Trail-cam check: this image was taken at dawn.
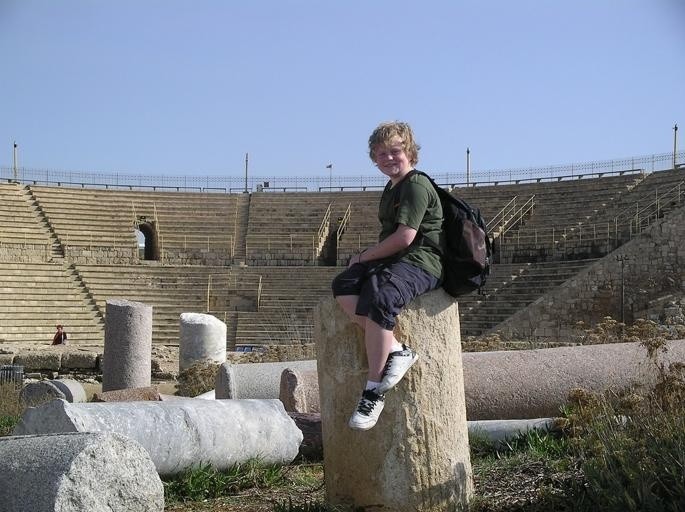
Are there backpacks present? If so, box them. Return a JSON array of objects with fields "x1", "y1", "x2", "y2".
[{"x1": 394, "y1": 170, "x2": 495, "y2": 298}]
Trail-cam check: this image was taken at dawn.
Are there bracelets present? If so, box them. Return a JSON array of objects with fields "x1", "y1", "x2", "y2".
[{"x1": 358, "y1": 255, "x2": 360, "y2": 264}]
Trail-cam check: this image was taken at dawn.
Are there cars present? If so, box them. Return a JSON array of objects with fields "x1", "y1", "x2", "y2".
[{"x1": 234, "y1": 343, "x2": 261, "y2": 353}]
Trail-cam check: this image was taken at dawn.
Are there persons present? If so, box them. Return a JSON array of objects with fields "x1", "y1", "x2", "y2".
[
  {"x1": 330, "y1": 118, "x2": 445, "y2": 429},
  {"x1": 51, "y1": 324, "x2": 67, "y2": 346}
]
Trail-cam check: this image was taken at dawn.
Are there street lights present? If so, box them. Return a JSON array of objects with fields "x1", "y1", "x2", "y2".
[
  {"x1": 673, "y1": 124, "x2": 678, "y2": 168},
  {"x1": 325, "y1": 163, "x2": 332, "y2": 192},
  {"x1": 466, "y1": 148, "x2": 470, "y2": 184},
  {"x1": 12, "y1": 142, "x2": 19, "y2": 181}
]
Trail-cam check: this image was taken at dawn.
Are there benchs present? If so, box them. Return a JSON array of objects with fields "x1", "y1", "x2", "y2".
[{"x1": 0, "y1": 167, "x2": 684, "y2": 351}]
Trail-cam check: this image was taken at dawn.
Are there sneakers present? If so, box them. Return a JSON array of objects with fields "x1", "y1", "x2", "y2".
[
  {"x1": 349, "y1": 390, "x2": 384, "y2": 431},
  {"x1": 379, "y1": 344, "x2": 419, "y2": 393}
]
